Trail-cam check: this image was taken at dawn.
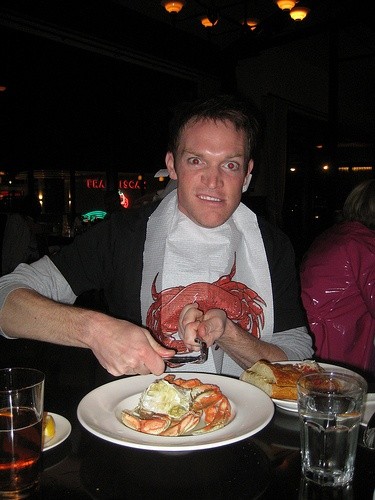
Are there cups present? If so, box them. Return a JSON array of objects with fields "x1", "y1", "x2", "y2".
[
  {"x1": 0, "y1": 368, "x2": 46, "y2": 494},
  {"x1": 297, "y1": 371, "x2": 368, "y2": 490}
]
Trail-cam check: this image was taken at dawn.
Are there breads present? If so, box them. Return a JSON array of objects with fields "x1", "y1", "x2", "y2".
[{"x1": 239, "y1": 359, "x2": 339, "y2": 401}]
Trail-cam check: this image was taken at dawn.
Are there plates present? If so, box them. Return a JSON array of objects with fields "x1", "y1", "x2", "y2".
[
  {"x1": 42, "y1": 412, "x2": 71, "y2": 452},
  {"x1": 76, "y1": 373, "x2": 275, "y2": 452},
  {"x1": 269, "y1": 361, "x2": 355, "y2": 412},
  {"x1": 360, "y1": 393, "x2": 375, "y2": 427}
]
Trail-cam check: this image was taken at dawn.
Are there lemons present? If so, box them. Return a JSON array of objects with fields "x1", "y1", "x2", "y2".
[{"x1": 43, "y1": 415, "x2": 55, "y2": 437}]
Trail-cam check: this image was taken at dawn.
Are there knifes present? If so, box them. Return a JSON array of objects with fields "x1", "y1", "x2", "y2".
[{"x1": 161, "y1": 356, "x2": 197, "y2": 363}]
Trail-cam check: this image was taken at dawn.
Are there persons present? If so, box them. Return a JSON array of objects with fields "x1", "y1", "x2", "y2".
[
  {"x1": 2, "y1": 194, "x2": 42, "y2": 275},
  {"x1": 0, "y1": 95, "x2": 314, "y2": 390},
  {"x1": 299, "y1": 179, "x2": 375, "y2": 374}
]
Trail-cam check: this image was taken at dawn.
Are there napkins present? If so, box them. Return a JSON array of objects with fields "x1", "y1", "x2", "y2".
[{"x1": 140, "y1": 190, "x2": 274, "y2": 378}]
